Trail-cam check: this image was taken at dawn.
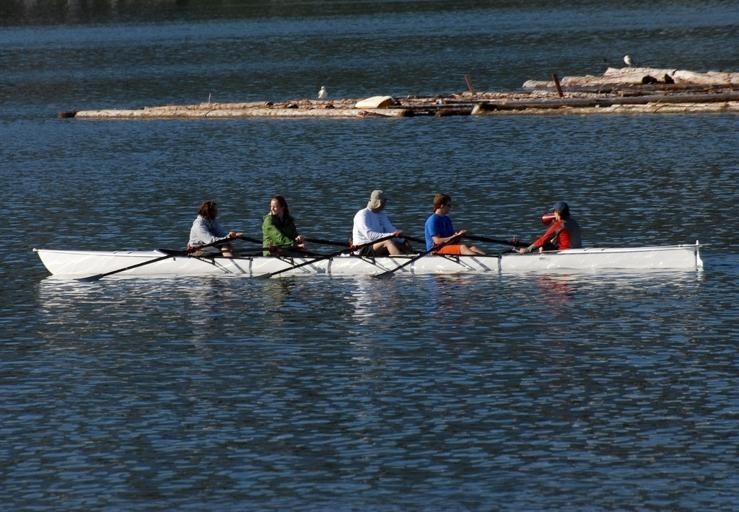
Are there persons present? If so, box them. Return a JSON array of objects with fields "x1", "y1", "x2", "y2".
[
  {"x1": 185, "y1": 199, "x2": 242, "y2": 258},
  {"x1": 424, "y1": 191, "x2": 488, "y2": 256},
  {"x1": 349, "y1": 188, "x2": 411, "y2": 257},
  {"x1": 512, "y1": 200, "x2": 582, "y2": 254},
  {"x1": 262, "y1": 195, "x2": 306, "y2": 256}
]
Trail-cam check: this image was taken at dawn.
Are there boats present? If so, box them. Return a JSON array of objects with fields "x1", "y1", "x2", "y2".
[{"x1": 26, "y1": 237, "x2": 705, "y2": 284}]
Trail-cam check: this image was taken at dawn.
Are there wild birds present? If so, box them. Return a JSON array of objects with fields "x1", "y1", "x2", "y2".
[
  {"x1": 318, "y1": 85, "x2": 328, "y2": 101},
  {"x1": 623, "y1": 54, "x2": 635, "y2": 67}
]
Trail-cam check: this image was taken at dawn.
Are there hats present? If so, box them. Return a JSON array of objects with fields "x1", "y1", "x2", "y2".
[
  {"x1": 369, "y1": 190, "x2": 385, "y2": 209},
  {"x1": 549, "y1": 202, "x2": 568, "y2": 210}
]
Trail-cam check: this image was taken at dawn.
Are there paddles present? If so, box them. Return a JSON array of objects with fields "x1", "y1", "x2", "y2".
[
  {"x1": 74, "y1": 232, "x2": 242, "y2": 282},
  {"x1": 369, "y1": 233, "x2": 461, "y2": 278},
  {"x1": 244, "y1": 234, "x2": 393, "y2": 279}
]
